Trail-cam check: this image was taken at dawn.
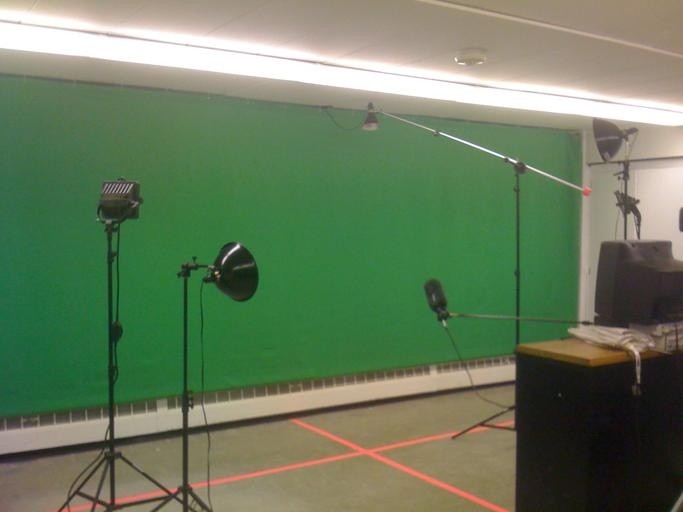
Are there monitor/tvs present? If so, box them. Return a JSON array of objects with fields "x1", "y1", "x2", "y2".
[{"x1": 594, "y1": 240, "x2": 683, "y2": 329}]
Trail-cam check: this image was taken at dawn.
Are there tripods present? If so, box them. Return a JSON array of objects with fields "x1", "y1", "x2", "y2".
[
  {"x1": 150, "y1": 263, "x2": 215, "y2": 512},
  {"x1": 452, "y1": 174, "x2": 520, "y2": 438},
  {"x1": 56, "y1": 220, "x2": 199, "y2": 512}
]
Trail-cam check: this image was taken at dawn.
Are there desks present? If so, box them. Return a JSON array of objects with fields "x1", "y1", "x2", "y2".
[{"x1": 513, "y1": 334, "x2": 683, "y2": 512}]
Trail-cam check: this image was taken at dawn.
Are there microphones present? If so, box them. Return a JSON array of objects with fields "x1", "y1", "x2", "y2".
[{"x1": 424, "y1": 280, "x2": 449, "y2": 321}]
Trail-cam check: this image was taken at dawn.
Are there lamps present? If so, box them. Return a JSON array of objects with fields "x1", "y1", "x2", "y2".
[
  {"x1": 146, "y1": 239, "x2": 258, "y2": 511},
  {"x1": 357, "y1": 102, "x2": 592, "y2": 442},
  {"x1": 588, "y1": 119, "x2": 637, "y2": 240},
  {"x1": 54, "y1": 175, "x2": 198, "y2": 512}
]
